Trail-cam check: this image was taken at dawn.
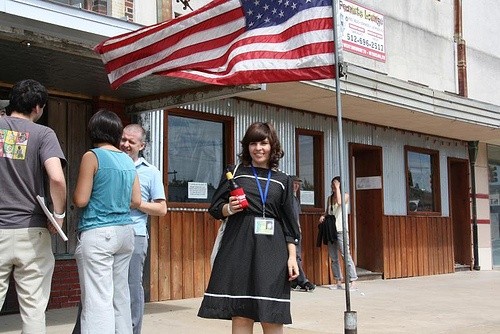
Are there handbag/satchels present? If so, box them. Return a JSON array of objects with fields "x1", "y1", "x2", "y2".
[{"x1": 209, "y1": 217, "x2": 228, "y2": 272}]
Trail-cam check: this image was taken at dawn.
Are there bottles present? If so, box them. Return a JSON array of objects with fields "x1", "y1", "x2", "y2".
[{"x1": 226, "y1": 172, "x2": 250, "y2": 214}]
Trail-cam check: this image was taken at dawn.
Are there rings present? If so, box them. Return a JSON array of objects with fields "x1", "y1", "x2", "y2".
[{"x1": 49, "y1": 225, "x2": 51, "y2": 227}]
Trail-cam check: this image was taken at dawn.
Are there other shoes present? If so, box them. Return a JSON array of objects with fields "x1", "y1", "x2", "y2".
[
  {"x1": 305, "y1": 281, "x2": 317, "y2": 291},
  {"x1": 290, "y1": 283, "x2": 306, "y2": 292},
  {"x1": 349, "y1": 281, "x2": 356, "y2": 290},
  {"x1": 337, "y1": 283, "x2": 345, "y2": 290}
]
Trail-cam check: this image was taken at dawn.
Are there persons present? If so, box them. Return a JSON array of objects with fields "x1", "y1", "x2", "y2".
[
  {"x1": 0, "y1": 80, "x2": 69, "y2": 334},
  {"x1": 288, "y1": 176, "x2": 316, "y2": 292},
  {"x1": 321, "y1": 176, "x2": 359, "y2": 291},
  {"x1": 197, "y1": 121, "x2": 300, "y2": 334},
  {"x1": 72, "y1": 110, "x2": 167, "y2": 334}
]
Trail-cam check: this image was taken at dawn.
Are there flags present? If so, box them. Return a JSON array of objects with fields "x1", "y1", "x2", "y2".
[{"x1": 92, "y1": 0, "x2": 335, "y2": 90}]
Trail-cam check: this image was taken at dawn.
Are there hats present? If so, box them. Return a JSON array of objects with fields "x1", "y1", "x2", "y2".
[{"x1": 291, "y1": 175, "x2": 303, "y2": 183}]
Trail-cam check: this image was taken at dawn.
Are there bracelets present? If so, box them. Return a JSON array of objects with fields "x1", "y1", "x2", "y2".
[
  {"x1": 227, "y1": 203, "x2": 234, "y2": 215},
  {"x1": 54, "y1": 211, "x2": 66, "y2": 219}
]
